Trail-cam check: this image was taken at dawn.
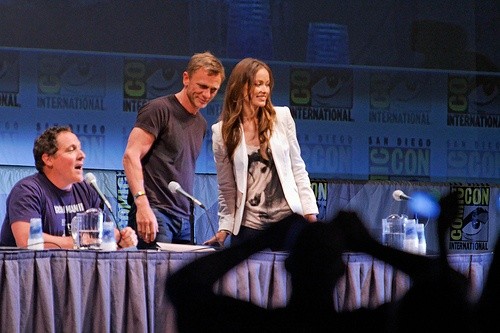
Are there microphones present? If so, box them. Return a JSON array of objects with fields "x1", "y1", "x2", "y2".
[
  {"x1": 393, "y1": 190, "x2": 416, "y2": 202},
  {"x1": 168, "y1": 181, "x2": 206, "y2": 210},
  {"x1": 84, "y1": 172, "x2": 112, "y2": 214}
]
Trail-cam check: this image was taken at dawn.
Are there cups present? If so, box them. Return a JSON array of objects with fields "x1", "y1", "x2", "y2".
[
  {"x1": 405, "y1": 219, "x2": 417, "y2": 254},
  {"x1": 418, "y1": 224, "x2": 427, "y2": 255},
  {"x1": 103, "y1": 221, "x2": 116, "y2": 249},
  {"x1": 27, "y1": 218, "x2": 44, "y2": 250}
]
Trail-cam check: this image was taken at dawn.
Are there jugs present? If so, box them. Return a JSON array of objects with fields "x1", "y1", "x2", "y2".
[
  {"x1": 381, "y1": 214, "x2": 408, "y2": 250},
  {"x1": 72, "y1": 209, "x2": 103, "y2": 250}
]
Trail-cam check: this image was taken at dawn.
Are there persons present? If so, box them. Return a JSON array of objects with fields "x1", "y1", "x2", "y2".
[
  {"x1": 1, "y1": 126, "x2": 138, "y2": 251},
  {"x1": 472, "y1": 228, "x2": 500, "y2": 333},
  {"x1": 123, "y1": 53, "x2": 225, "y2": 249},
  {"x1": 203, "y1": 57, "x2": 320, "y2": 249},
  {"x1": 165, "y1": 210, "x2": 470, "y2": 333}
]
